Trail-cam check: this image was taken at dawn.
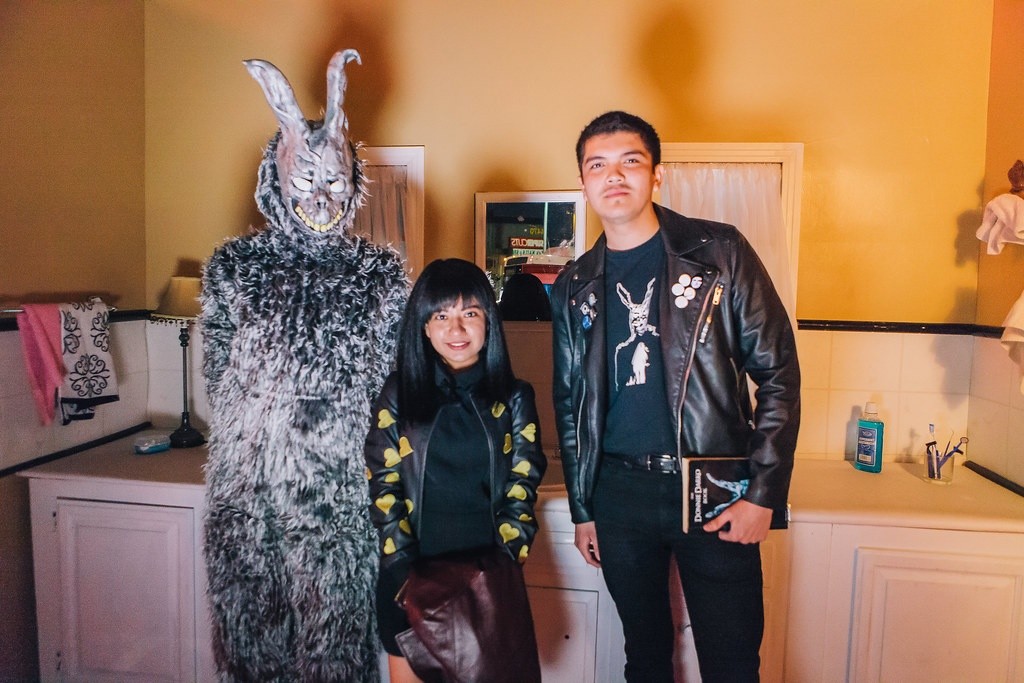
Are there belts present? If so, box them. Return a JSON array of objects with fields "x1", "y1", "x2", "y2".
[{"x1": 600, "y1": 453, "x2": 679, "y2": 474}]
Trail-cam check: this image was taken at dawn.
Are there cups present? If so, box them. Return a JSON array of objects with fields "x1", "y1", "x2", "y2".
[{"x1": 923, "y1": 452, "x2": 956, "y2": 484}]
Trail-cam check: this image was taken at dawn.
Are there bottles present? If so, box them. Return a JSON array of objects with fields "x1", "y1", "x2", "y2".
[{"x1": 853, "y1": 402, "x2": 885, "y2": 473}]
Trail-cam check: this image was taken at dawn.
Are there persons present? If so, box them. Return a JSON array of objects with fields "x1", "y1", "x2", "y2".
[
  {"x1": 493, "y1": 272, "x2": 552, "y2": 322},
  {"x1": 546, "y1": 109, "x2": 801, "y2": 683},
  {"x1": 363, "y1": 258, "x2": 547, "y2": 683},
  {"x1": 202, "y1": 46, "x2": 414, "y2": 683}
]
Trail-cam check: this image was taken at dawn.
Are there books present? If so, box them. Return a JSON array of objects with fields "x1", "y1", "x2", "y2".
[{"x1": 681, "y1": 457, "x2": 789, "y2": 533}]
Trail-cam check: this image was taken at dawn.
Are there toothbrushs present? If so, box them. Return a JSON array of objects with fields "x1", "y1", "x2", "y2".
[{"x1": 928, "y1": 423, "x2": 938, "y2": 480}]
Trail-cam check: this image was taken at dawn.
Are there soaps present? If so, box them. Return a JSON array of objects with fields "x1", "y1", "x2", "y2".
[{"x1": 134, "y1": 435, "x2": 172, "y2": 454}]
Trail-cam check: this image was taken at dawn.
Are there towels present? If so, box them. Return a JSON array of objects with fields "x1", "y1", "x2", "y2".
[
  {"x1": 999, "y1": 290, "x2": 1024, "y2": 395},
  {"x1": 975, "y1": 193, "x2": 1024, "y2": 256},
  {"x1": 16, "y1": 296, "x2": 120, "y2": 426}
]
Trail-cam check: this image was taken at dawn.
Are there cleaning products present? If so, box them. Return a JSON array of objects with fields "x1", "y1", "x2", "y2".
[{"x1": 854, "y1": 402, "x2": 884, "y2": 473}]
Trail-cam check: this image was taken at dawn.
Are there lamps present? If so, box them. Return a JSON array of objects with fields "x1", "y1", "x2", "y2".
[{"x1": 151, "y1": 276, "x2": 207, "y2": 449}]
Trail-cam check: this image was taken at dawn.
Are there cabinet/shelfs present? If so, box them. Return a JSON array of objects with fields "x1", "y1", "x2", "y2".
[{"x1": 28, "y1": 482, "x2": 1024, "y2": 683}]
